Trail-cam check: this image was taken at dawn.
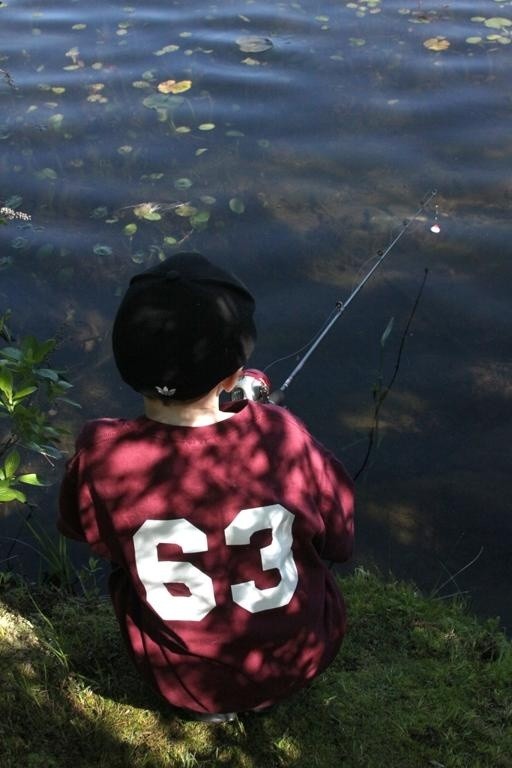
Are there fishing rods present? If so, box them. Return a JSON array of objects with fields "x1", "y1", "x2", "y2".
[{"x1": 235, "y1": 187, "x2": 439, "y2": 404}]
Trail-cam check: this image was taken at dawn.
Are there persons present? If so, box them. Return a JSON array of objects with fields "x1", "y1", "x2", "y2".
[{"x1": 56, "y1": 253, "x2": 356, "y2": 723}]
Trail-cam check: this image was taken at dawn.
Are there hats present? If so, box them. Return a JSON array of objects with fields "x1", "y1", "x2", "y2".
[{"x1": 112, "y1": 251, "x2": 260, "y2": 404}]
